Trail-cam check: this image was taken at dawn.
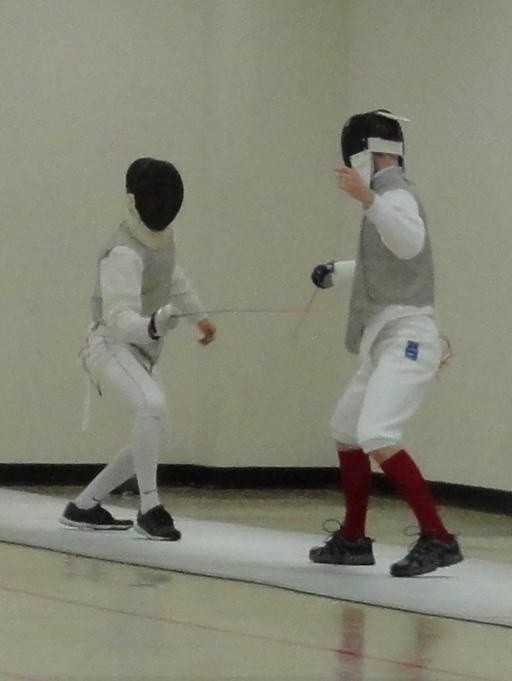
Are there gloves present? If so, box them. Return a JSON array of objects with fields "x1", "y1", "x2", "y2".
[
  {"x1": 311, "y1": 261, "x2": 334, "y2": 290},
  {"x1": 148, "y1": 303, "x2": 179, "y2": 339}
]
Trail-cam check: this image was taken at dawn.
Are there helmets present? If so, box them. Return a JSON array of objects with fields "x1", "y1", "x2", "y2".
[
  {"x1": 126, "y1": 157, "x2": 184, "y2": 249},
  {"x1": 341, "y1": 109, "x2": 406, "y2": 189}
]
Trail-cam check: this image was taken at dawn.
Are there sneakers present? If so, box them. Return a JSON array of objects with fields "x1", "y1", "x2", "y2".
[
  {"x1": 309, "y1": 519, "x2": 376, "y2": 565},
  {"x1": 59, "y1": 501, "x2": 134, "y2": 530},
  {"x1": 391, "y1": 526, "x2": 463, "y2": 577},
  {"x1": 137, "y1": 504, "x2": 181, "y2": 541}
]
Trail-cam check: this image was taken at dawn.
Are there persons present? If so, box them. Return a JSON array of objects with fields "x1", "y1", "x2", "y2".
[
  {"x1": 305, "y1": 106, "x2": 466, "y2": 579},
  {"x1": 331, "y1": 600, "x2": 453, "y2": 681},
  {"x1": 55, "y1": 154, "x2": 214, "y2": 548}
]
